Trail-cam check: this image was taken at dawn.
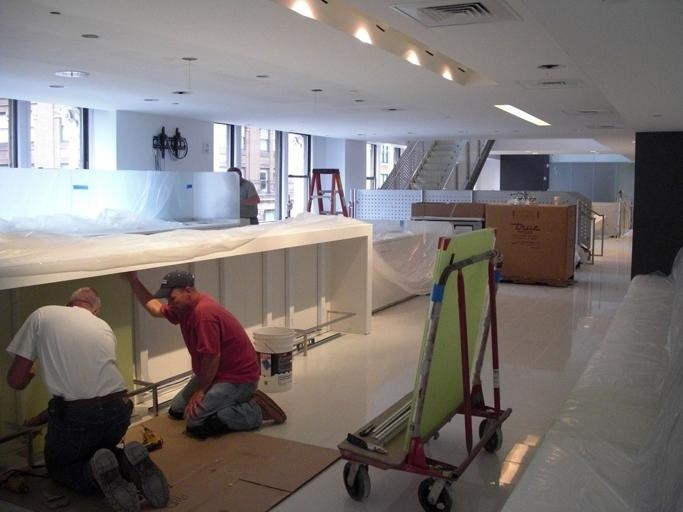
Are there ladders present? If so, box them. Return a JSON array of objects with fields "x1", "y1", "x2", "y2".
[{"x1": 307, "y1": 168, "x2": 348, "y2": 217}]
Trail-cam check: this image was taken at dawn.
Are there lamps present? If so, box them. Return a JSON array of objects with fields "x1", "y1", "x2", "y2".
[{"x1": 147, "y1": 123, "x2": 189, "y2": 159}]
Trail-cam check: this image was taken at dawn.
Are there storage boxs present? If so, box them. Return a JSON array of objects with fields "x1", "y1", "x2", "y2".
[{"x1": 483, "y1": 200, "x2": 577, "y2": 286}]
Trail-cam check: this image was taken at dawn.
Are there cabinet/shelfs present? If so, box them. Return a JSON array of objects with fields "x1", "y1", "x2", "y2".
[{"x1": 366, "y1": 216, "x2": 451, "y2": 317}]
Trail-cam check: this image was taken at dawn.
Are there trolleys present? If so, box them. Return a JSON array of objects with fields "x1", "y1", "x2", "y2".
[{"x1": 336, "y1": 248, "x2": 513, "y2": 511}]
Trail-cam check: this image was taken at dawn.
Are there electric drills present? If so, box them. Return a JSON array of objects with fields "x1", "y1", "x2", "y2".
[{"x1": 141, "y1": 423, "x2": 163, "y2": 452}]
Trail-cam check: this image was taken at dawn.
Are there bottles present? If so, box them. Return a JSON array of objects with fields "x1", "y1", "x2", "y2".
[{"x1": 0, "y1": 472, "x2": 30, "y2": 494}]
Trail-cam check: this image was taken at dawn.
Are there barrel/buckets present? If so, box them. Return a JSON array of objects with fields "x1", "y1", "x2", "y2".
[{"x1": 252, "y1": 326, "x2": 297, "y2": 394}]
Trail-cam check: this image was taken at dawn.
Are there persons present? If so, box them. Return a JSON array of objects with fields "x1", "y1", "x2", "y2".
[
  {"x1": 5, "y1": 287, "x2": 169, "y2": 512},
  {"x1": 228, "y1": 167, "x2": 260, "y2": 224},
  {"x1": 122, "y1": 271, "x2": 287, "y2": 440}
]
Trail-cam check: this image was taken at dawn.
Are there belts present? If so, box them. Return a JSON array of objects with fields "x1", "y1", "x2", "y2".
[{"x1": 66, "y1": 395, "x2": 118, "y2": 408}]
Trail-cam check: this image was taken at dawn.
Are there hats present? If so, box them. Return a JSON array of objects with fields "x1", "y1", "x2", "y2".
[{"x1": 152, "y1": 271, "x2": 196, "y2": 298}]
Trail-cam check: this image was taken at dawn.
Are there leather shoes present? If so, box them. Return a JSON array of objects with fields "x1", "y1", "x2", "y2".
[
  {"x1": 88, "y1": 448, "x2": 142, "y2": 511},
  {"x1": 121, "y1": 441, "x2": 170, "y2": 509},
  {"x1": 251, "y1": 388, "x2": 287, "y2": 423}
]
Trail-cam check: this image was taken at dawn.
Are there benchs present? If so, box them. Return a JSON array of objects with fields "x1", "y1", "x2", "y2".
[{"x1": 498, "y1": 246, "x2": 683, "y2": 512}]
